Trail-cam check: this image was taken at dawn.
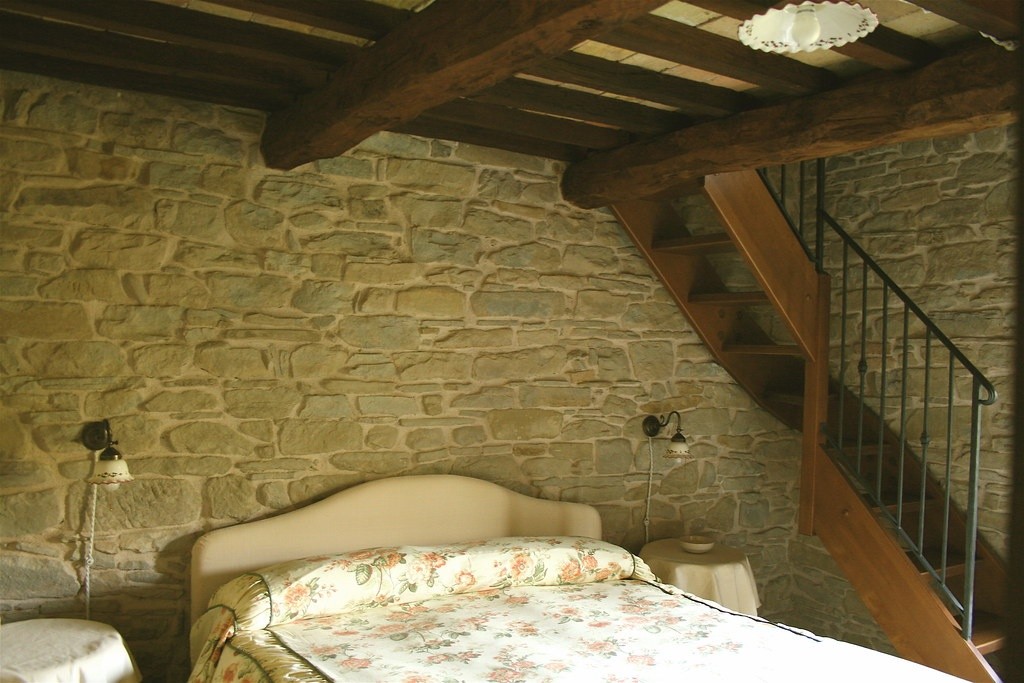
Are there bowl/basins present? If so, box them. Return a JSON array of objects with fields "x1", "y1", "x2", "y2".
[{"x1": 678, "y1": 536, "x2": 717, "y2": 554}]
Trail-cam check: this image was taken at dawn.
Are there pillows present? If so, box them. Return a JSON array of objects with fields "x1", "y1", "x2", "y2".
[{"x1": 186, "y1": 536, "x2": 657, "y2": 683}]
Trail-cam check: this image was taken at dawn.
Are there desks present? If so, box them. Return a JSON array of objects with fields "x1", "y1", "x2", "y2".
[
  {"x1": 637, "y1": 537, "x2": 761, "y2": 618},
  {"x1": 0, "y1": 618, "x2": 146, "y2": 683}
]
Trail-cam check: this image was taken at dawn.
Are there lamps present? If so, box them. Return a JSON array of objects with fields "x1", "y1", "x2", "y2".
[
  {"x1": 83, "y1": 418, "x2": 135, "y2": 491},
  {"x1": 737, "y1": 0, "x2": 879, "y2": 57},
  {"x1": 643, "y1": 411, "x2": 697, "y2": 462}
]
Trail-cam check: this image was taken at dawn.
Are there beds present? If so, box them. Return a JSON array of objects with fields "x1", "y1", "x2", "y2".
[{"x1": 186, "y1": 473, "x2": 969, "y2": 682}]
